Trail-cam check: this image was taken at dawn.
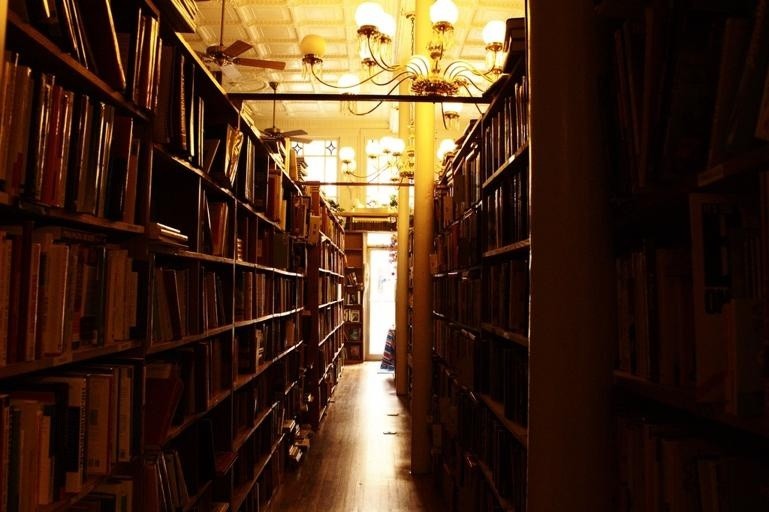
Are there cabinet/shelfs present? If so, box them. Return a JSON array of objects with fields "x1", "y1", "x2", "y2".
[
  {"x1": 408, "y1": 0, "x2": 769, "y2": 512},
  {"x1": 0, "y1": 0, "x2": 366, "y2": 512}
]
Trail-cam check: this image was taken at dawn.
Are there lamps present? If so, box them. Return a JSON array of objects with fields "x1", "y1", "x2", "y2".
[
  {"x1": 340, "y1": 107, "x2": 458, "y2": 192},
  {"x1": 300, "y1": 0, "x2": 507, "y2": 131}
]
